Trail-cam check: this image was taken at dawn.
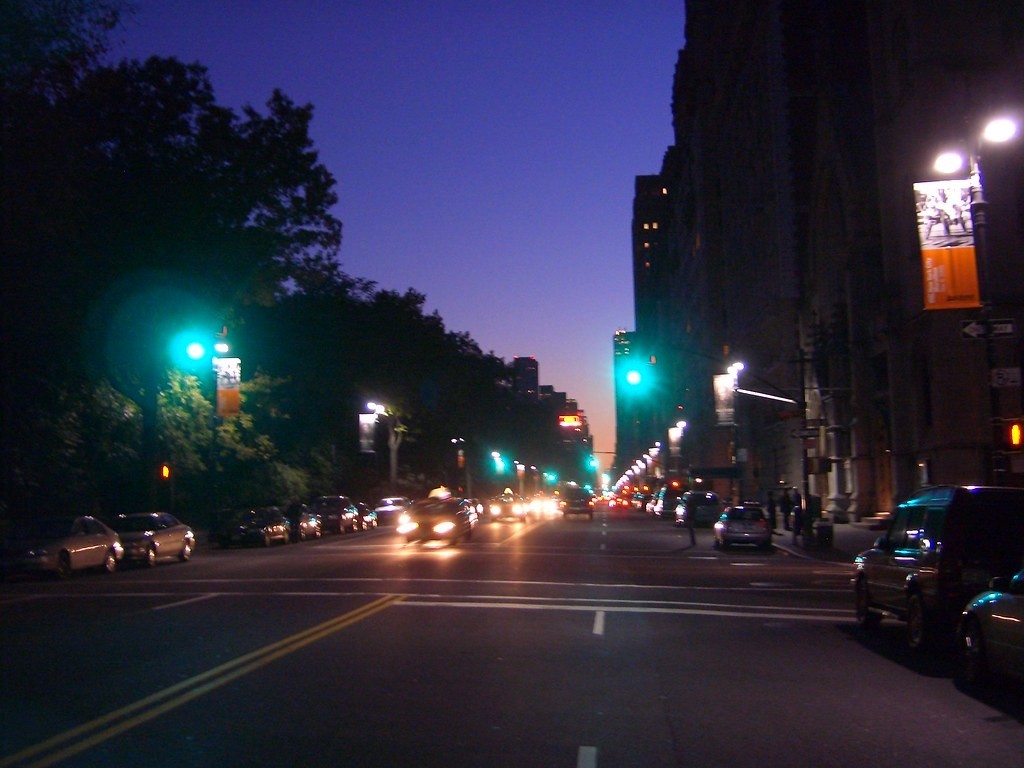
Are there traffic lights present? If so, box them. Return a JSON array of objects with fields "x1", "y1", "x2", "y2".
[
  {"x1": 159, "y1": 461, "x2": 171, "y2": 480},
  {"x1": 1004, "y1": 416, "x2": 1023, "y2": 451}
]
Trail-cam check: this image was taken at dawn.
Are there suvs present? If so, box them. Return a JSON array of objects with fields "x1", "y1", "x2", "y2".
[
  {"x1": 311, "y1": 494, "x2": 359, "y2": 536},
  {"x1": 850, "y1": 483, "x2": 1023, "y2": 654},
  {"x1": 562, "y1": 488, "x2": 596, "y2": 521}
]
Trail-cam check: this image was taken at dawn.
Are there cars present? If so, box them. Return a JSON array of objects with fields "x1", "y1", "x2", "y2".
[
  {"x1": 217, "y1": 505, "x2": 292, "y2": 549},
  {"x1": 354, "y1": 502, "x2": 378, "y2": 532},
  {"x1": 678, "y1": 489, "x2": 724, "y2": 529},
  {"x1": 611, "y1": 484, "x2": 685, "y2": 520},
  {"x1": 12, "y1": 515, "x2": 125, "y2": 582},
  {"x1": 713, "y1": 505, "x2": 771, "y2": 553},
  {"x1": 281, "y1": 503, "x2": 325, "y2": 543},
  {"x1": 958, "y1": 568, "x2": 1024, "y2": 686},
  {"x1": 475, "y1": 491, "x2": 559, "y2": 522},
  {"x1": 375, "y1": 497, "x2": 410, "y2": 524},
  {"x1": 400, "y1": 496, "x2": 478, "y2": 546},
  {"x1": 114, "y1": 513, "x2": 197, "y2": 568}
]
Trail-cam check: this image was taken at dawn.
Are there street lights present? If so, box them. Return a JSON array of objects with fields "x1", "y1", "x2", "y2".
[{"x1": 931, "y1": 113, "x2": 1017, "y2": 484}]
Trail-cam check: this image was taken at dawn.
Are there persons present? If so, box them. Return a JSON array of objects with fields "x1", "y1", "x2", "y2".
[
  {"x1": 767, "y1": 490, "x2": 776, "y2": 529},
  {"x1": 683, "y1": 496, "x2": 697, "y2": 545},
  {"x1": 780, "y1": 486, "x2": 802, "y2": 531}
]
